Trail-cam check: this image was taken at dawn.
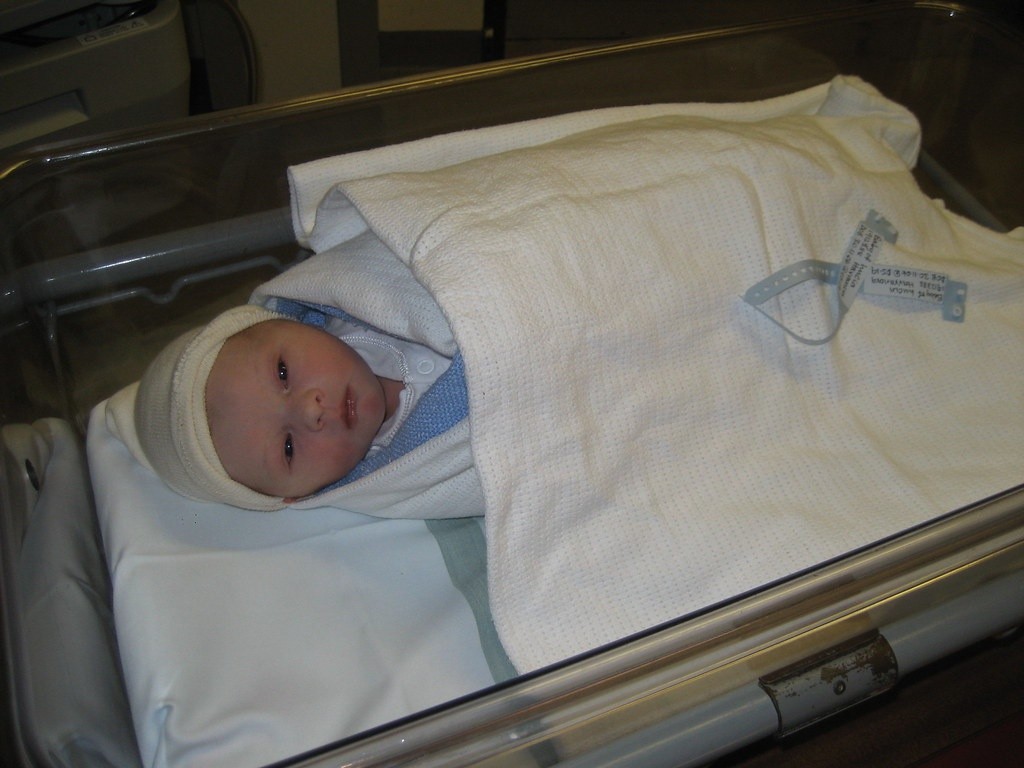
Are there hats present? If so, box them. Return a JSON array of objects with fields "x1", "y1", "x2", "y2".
[{"x1": 105, "y1": 305, "x2": 301, "y2": 512}]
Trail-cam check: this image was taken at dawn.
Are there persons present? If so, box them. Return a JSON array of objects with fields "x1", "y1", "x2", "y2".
[{"x1": 102, "y1": 230, "x2": 486, "y2": 523}]
